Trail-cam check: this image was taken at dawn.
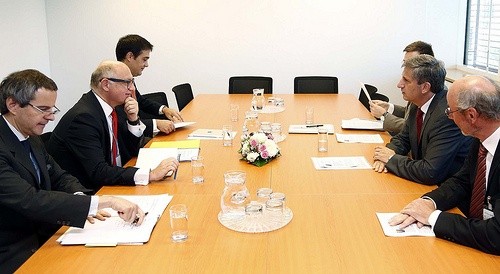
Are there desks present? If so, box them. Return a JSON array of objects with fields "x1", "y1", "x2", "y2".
[{"x1": 13, "y1": 94, "x2": 500, "y2": 274}]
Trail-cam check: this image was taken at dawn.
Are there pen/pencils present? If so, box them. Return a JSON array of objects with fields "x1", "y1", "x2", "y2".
[
  {"x1": 306, "y1": 124, "x2": 324, "y2": 128},
  {"x1": 132, "y1": 212, "x2": 149, "y2": 224},
  {"x1": 227, "y1": 132, "x2": 230, "y2": 136},
  {"x1": 174, "y1": 153, "x2": 181, "y2": 179}
]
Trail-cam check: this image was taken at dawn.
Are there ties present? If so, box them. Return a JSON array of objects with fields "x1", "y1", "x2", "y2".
[
  {"x1": 469, "y1": 144, "x2": 488, "y2": 222},
  {"x1": 111, "y1": 110, "x2": 118, "y2": 166},
  {"x1": 416, "y1": 106, "x2": 424, "y2": 144}
]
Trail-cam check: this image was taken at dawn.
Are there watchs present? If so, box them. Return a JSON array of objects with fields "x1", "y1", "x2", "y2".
[{"x1": 380, "y1": 112, "x2": 389, "y2": 122}]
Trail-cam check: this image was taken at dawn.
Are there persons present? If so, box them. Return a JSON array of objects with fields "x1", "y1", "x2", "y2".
[
  {"x1": 0, "y1": 69, "x2": 145, "y2": 274},
  {"x1": 369, "y1": 41, "x2": 435, "y2": 135},
  {"x1": 46, "y1": 60, "x2": 179, "y2": 195},
  {"x1": 387, "y1": 74, "x2": 500, "y2": 257},
  {"x1": 372, "y1": 54, "x2": 474, "y2": 188},
  {"x1": 115, "y1": 34, "x2": 184, "y2": 138}
]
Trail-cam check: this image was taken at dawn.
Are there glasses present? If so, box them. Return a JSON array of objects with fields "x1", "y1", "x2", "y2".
[
  {"x1": 445, "y1": 106, "x2": 462, "y2": 117},
  {"x1": 28, "y1": 102, "x2": 60, "y2": 116},
  {"x1": 99, "y1": 76, "x2": 135, "y2": 89}
]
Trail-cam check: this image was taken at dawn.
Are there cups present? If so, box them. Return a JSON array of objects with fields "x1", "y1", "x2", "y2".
[
  {"x1": 231, "y1": 104, "x2": 239, "y2": 122},
  {"x1": 170, "y1": 203, "x2": 188, "y2": 241},
  {"x1": 318, "y1": 129, "x2": 328, "y2": 152},
  {"x1": 192, "y1": 156, "x2": 206, "y2": 184},
  {"x1": 245, "y1": 187, "x2": 286, "y2": 225},
  {"x1": 261, "y1": 122, "x2": 282, "y2": 140},
  {"x1": 268, "y1": 96, "x2": 284, "y2": 112},
  {"x1": 306, "y1": 106, "x2": 314, "y2": 125},
  {"x1": 223, "y1": 125, "x2": 232, "y2": 147}
]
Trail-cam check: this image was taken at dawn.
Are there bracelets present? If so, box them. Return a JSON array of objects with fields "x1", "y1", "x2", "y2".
[{"x1": 128, "y1": 117, "x2": 140, "y2": 126}]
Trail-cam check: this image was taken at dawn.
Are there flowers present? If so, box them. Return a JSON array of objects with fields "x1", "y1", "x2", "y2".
[{"x1": 239, "y1": 130, "x2": 283, "y2": 168}]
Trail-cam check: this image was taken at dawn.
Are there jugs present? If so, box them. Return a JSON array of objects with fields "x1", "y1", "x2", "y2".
[
  {"x1": 222, "y1": 170, "x2": 251, "y2": 219},
  {"x1": 250, "y1": 88, "x2": 265, "y2": 113},
  {"x1": 244, "y1": 112, "x2": 261, "y2": 133}
]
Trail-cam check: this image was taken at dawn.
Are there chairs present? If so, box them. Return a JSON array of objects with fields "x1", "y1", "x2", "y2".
[
  {"x1": 293, "y1": 76, "x2": 339, "y2": 95},
  {"x1": 229, "y1": 76, "x2": 273, "y2": 95},
  {"x1": 359, "y1": 84, "x2": 390, "y2": 113},
  {"x1": 171, "y1": 84, "x2": 193, "y2": 111}
]
